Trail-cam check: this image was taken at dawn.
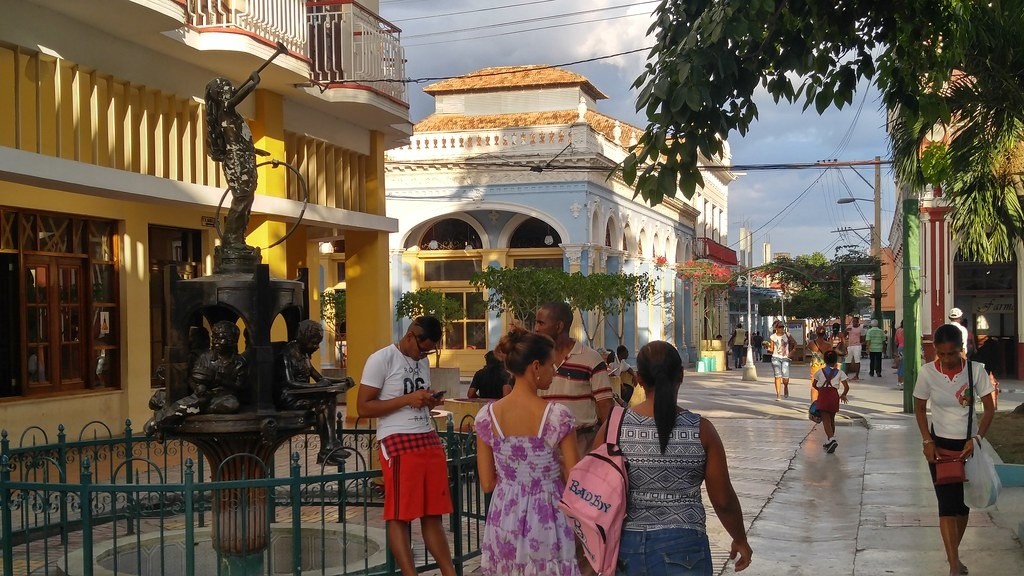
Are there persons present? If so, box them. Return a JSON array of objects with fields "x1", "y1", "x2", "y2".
[
  {"x1": 534, "y1": 301, "x2": 615, "y2": 462},
  {"x1": 474, "y1": 321, "x2": 580, "y2": 576},
  {"x1": 273, "y1": 320, "x2": 356, "y2": 466},
  {"x1": 143, "y1": 321, "x2": 249, "y2": 444},
  {"x1": 913, "y1": 325, "x2": 994, "y2": 576},
  {"x1": 357, "y1": 315, "x2": 459, "y2": 576},
  {"x1": 813, "y1": 352, "x2": 850, "y2": 454},
  {"x1": 716, "y1": 323, "x2": 764, "y2": 371},
  {"x1": 768, "y1": 321, "x2": 797, "y2": 401},
  {"x1": 805, "y1": 316, "x2": 903, "y2": 404},
  {"x1": 467, "y1": 351, "x2": 516, "y2": 400},
  {"x1": 204, "y1": 70, "x2": 271, "y2": 250},
  {"x1": 597, "y1": 345, "x2": 638, "y2": 392},
  {"x1": 14, "y1": 341, "x2": 37, "y2": 396},
  {"x1": 592, "y1": 341, "x2": 753, "y2": 576},
  {"x1": 948, "y1": 308, "x2": 978, "y2": 359}
]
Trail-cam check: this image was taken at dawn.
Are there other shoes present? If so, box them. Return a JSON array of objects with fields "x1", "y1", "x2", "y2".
[
  {"x1": 776, "y1": 387, "x2": 788, "y2": 401},
  {"x1": 897, "y1": 381, "x2": 904, "y2": 385},
  {"x1": 869, "y1": 371, "x2": 882, "y2": 377},
  {"x1": 846, "y1": 374, "x2": 859, "y2": 381},
  {"x1": 823, "y1": 440, "x2": 838, "y2": 454},
  {"x1": 947, "y1": 558, "x2": 969, "y2": 575}
]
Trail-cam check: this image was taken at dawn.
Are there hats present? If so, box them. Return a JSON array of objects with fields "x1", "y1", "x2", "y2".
[
  {"x1": 596, "y1": 348, "x2": 612, "y2": 356},
  {"x1": 949, "y1": 308, "x2": 964, "y2": 319}
]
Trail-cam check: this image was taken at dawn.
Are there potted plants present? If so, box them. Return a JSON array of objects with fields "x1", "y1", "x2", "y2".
[
  {"x1": 395, "y1": 285, "x2": 465, "y2": 400},
  {"x1": 605, "y1": 272, "x2": 657, "y2": 387},
  {"x1": 562, "y1": 266, "x2": 622, "y2": 400},
  {"x1": 320, "y1": 288, "x2": 348, "y2": 406}
]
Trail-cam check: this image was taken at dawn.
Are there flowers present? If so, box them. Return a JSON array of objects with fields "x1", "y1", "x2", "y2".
[
  {"x1": 674, "y1": 260, "x2": 740, "y2": 350},
  {"x1": 653, "y1": 255, "x2": 669, "y2": 272}
]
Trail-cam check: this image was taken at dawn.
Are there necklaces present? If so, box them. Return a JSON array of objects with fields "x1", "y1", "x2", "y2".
[{"x1": 398, "y1": 342, "x2": 419, "y2": 375}]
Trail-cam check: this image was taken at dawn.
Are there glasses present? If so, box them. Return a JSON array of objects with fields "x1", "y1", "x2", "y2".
[
  {"x1": 778, "y1": 325, "x2": 784, "y2": 329},
  {"x1": 411, "y1": 331, "x2": 438, "y2": 356}
]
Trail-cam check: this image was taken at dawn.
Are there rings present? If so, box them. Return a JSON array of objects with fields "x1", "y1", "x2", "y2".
[
  {"x1": 749, "y1": 560, "x2": 752, "y2": 563},
  {"x1": 926, "y1": 456, "x2": 929, "y2": 460}
]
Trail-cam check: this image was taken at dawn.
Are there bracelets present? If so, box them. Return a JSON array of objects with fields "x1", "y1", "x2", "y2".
[
  {"x1": 792, "y1": 349, "x2": 796, "y2": 352},
  {"x1": 922, "y1": 440, "x2": 935, "y2": 446}
]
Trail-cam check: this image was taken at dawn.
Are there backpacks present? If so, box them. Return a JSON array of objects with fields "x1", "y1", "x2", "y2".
[
  {"x1": 815, "y1": 368, "x2": 839, "y2": 412},
  {"x1": 557, "y1": 406, "x2": 629, "y2": 576}
]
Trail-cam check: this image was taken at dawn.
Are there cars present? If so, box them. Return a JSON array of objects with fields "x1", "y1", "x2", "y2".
[{"x1": 862, "y1": 315, "x2": 871, "y2": 321}]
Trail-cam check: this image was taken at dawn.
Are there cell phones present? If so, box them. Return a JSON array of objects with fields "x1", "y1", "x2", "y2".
[{"x1": 432, "y1": 390, "x2": 448, "y2": 397}]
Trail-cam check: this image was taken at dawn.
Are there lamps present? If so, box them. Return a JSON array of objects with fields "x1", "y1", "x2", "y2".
[
  {"x1": 543, "y1": 224, "x2": 554, "y2": 247},
  {"x1": 463, "y1": 223, "x2": 473, "y2": 250},
  {"x1": 429, "y1": 225, "x2": 439, "y2": 250},
  {"x1": 319, "y1": 241, "x2": 334, "y2": 254}
]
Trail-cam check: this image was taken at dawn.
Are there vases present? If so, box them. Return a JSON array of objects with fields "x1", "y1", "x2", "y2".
[{"x1": 700, "y1": 350, "x2": 725, "y2": 372}]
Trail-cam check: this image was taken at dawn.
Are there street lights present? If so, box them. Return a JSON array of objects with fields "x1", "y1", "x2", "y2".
[
  {"x1": 736, "y1": 270, "x2": 758, "y2": 380},
  {"x1": 837, "y1": 198, "x2": 884, "y2": 371},
  {"x1": 773, "y1": 295, "x2": 792, "y2": 323}
]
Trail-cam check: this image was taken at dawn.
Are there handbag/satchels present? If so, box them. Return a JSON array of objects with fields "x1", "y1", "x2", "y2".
[
  {"x1": 963, "y1": 435, "x2": 1003, "y2": 511},
  {"x1": 809, "y1": 400, "x2": 823, "y2": 424},
  {"x1": 834, "y1": 342, "x2": 848, "y2": 356},
  {"x1": 934, "y1": 457, "x2": 969, "y2": 484},
  {"x1": 788, "y1": 339, "x2": 794, "y2": 352}
]
